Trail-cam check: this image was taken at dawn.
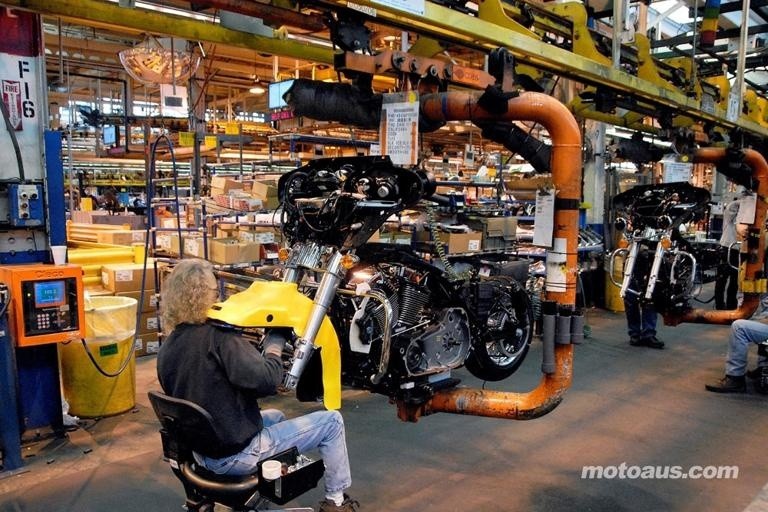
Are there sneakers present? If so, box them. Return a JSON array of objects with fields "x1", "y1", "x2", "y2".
[
  {"x1": 630, "y1": 335, "x2": 664, "y2": 347},
  {"x1": 704, "y1": 376, "x2": 747, "y2": 392},
  {"x1": 320, "y1": 494, "x2": 360, "y2": 512}
]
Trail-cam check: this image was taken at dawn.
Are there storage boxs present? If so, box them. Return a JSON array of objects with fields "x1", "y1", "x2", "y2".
[
  {"x1": 416, "y1": 231, "x2": 482, "y2": 255},
  {"x1": 97, "y1": 173, "x2": 287, "y2": 358}
]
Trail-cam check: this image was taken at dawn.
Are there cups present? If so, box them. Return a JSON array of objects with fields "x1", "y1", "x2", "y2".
[
  {"x1": 50, "y1": 245, "x2": 68, "y2": 266},
  {"x1": 262, "y1": 460, "x2": 281, "y2": 480}
]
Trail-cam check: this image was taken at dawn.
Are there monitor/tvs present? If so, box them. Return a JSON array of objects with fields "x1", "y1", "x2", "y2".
[
  {"x1": 103, "y1": 126, "x2": 117, "y2": 145},
  {"x1": 268, "y1": 79, "x2": 296, "y2": 109}
]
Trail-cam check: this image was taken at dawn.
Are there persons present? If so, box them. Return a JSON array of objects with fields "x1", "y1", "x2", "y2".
[
  {"x1": 705, "y1": 317, "x2": 768, "y2": 393},
  {"x1": 156, "y1": 259, "x2": 360, "y2": 512},
  {"x1": 715, "y1": 192, "x2": 742, "y2": 311},
  {"x1": 104, "y1": 188, "x2": 115, "y2": 216},
  {"x1": 74, "y1": 187, "x2": 81, "y2": 211},
  {"x1": 621, "y1": 229, "x2": 665, "y2": 348}
]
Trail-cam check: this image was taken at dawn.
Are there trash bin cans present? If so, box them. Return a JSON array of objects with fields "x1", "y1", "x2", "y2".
[
  {"x1": 57, "y1": 295, "x2": 138, "y2": 419},
  {"x1": 604, "y1": 254, "x2": 626, "y2": 312}
]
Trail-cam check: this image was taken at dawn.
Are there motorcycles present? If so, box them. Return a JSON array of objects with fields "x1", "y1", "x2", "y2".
[
  {"x1": 606, "y1": 181, "x2": 730, "y2": 312},
  {"x1": 206, "y1": 154, "x2": 535, "y2": 402}
]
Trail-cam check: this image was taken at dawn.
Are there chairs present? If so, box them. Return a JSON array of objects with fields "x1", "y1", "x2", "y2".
[{"x1": 147, "y1": 390, "x2": 325, "y2": 512}]
